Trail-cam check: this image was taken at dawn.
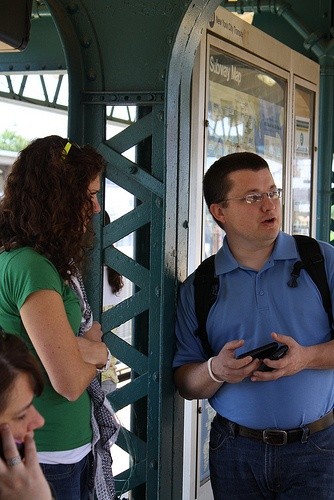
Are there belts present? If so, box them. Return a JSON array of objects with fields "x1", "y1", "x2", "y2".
[{"x1": 214, "y1": 413, "x2": 334, "y2": 444}]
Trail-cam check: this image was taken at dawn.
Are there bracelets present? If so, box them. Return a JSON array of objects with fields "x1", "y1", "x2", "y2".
[
  {"x1": 207, "y1": 356, "x2": 225, "y2": 383},
  {"x1": 96, "y1": 347, "x2": 111, "y2": 373}
]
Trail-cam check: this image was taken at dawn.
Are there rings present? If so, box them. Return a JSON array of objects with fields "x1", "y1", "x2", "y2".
[{"x1": 6, "y1": 456, "x2": 23, "y2": 465}]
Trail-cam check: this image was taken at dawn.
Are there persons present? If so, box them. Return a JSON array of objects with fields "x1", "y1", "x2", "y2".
[
  {"x1": 0, "y1": 135, "x2": 120, "y2": 499},
  {"x1": 170, "y1": 151, "x2": 334, "y2": 500},
  {"x1": 101, "y1": 206, "x2": 126, "y2": 399},
  {"x1": 0, "y1": 327, "x2": 55, "y2": 499}
]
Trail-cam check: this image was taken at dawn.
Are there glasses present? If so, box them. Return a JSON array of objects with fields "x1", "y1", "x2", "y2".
[{"x1": 217, "y1": 188, "x2": 281, "y2": 205}]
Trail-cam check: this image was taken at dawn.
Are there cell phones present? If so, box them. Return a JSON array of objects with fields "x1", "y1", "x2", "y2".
[
  {"x1": 270, "y1": 345, "x2": 288, "y2": 360},
  {"x1": 235, "y1": 342, "x2": 278, "y2": 364},
  {"x1": 0, "y1": 437, "x2": 25, "y2": 461}
]
